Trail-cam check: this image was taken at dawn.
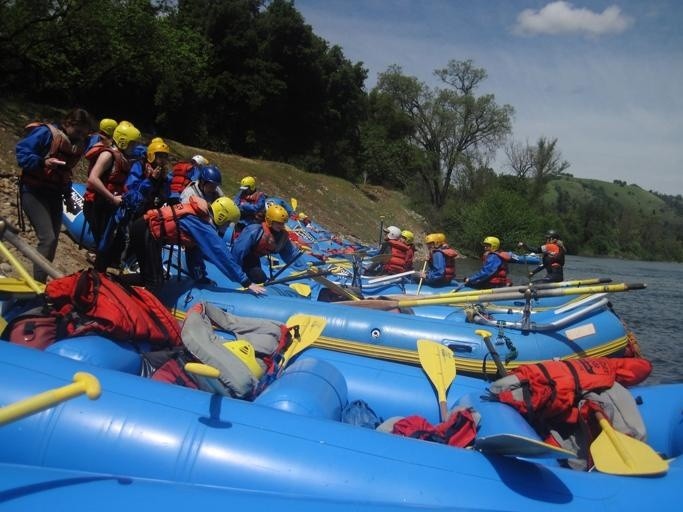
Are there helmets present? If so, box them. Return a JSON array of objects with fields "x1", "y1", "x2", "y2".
[
  {"x1": 439, "y1": 234, "x2": 445, "y2": 246},
  {"x1": 210, "y1": 197, "x2": 240, "y2": 227},
  {"x1": 544, "y1": 229, "x2": 559, "y2": 240},
  {"x1": 238, "y1": 175, "x2": 255, "y2": 192},
  {"x1": 113, "y1": 127, "x2": 142, "y2": 152},
  {"x1": 191, "y1": 153, "x2": 203, "y2": 166},
  {"x1": 97, "y1": 117, "x2": 116, "y2": 139},
  {"x1": 198, "y1": 166, "x2": 221, "y2": 185},
  {"x1": 149, "y1": 135, "x2": 165, "y2": 144},
  {"x1": 119, "y1": 121, "x2": 132, "y2": 127},
  {"x1": 481, "y1": 235, "x2": 501, "y2": 252},
  {"x1": 264, "y1": 204, "x2": 289, "y2": 227},
  {"x1": 145, "y1": 144, "x2": 170, "y2": 163},
  {"x1": 381, "y1": 224, "x2": 400, "y2": 242},
  {"x1": 401, "y1": 231, "x2": 413, "y2": 244},
  {"x1": 424, "y1": 233, "x2": 439, "y2": 250}
]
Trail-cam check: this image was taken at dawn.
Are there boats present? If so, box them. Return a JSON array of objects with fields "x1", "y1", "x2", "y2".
[
  {"x1": 0, "y1": 272, "x2": 683, "y2": 507},
  {"x1": 175, "y1": 279, "x2": 633, "y2": 379},
  {"x1": 243, "y1": 191, "x2": 474, "y2": 293},
  {"x1": 57, "y1": 178, "x2": 240, "y2": 287},
  {"x1": 497, "y1": 251, "x2": 545, "y2": 265}
]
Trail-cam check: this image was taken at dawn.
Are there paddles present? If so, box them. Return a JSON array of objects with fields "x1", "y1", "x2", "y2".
[
  {"x1": 328, "y1": 283, "x2": 647, "y2": 312},
  {"x1": 466, "y1": 431, "x2": 579, "y2": 469},
  {"x1": 362, "y1": 278, "x2": 612, "y2": 301},
  {"x1": 306, "y1": 268, "x2": 359, "y2": 302},
  {"x1": 283, "y1": 282, "x2": 312, "y2": 297},
  {"x1": 415, "y1": 336, "x2": 457, "y2": 429},
  {"x1": 0, "y1": 276, "x2": 47, "y2": 293},
  {"x1": 588, "y1": 396, "x2": 670, "y2": 478},
  {"x1": 274, "y1": 312, "x2": 328, "y2": 377},
  {"x1": 291, "y1": 197, "x2": 299, "y2": 212}
]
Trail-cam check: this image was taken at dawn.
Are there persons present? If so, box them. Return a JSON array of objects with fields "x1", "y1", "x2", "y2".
[
  {"x1": 362, "y1": 226, "x2": 459, "y2": 288},
  {"x1": 16, "y1": 109, "x2": 323, "y2": 307},
  {"x1": 464, "y1": 236, "x2": 513, "y2": 290},
  {"x1": 517, "y1": 230, "x2": 567, "y2": 284}
]
